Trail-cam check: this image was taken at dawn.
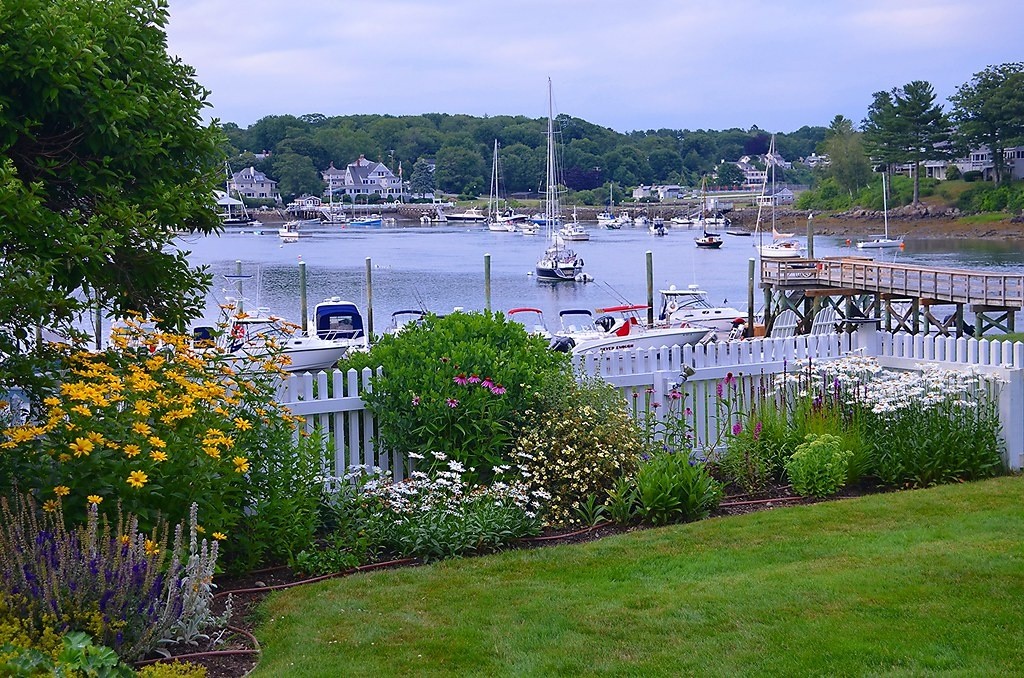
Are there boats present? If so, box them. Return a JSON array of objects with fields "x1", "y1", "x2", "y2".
[
  {"x1": 597, "y1": 184, "x2": 732, "y2": 237},
  {"x1": 381, "y1": 286, "x2": 444, "y2": 339},
  {"x1": 308, "y1": 296, "x2": 369, "y2": 361},
  {"x1": 247, "y1": 220, "x2": 264, "y2": 225},
  {"x1": 856, "y1": 172, "x2": 906, "y2": 248},
  {"x1": 106, "y1": 265, "x2": 351, "y2": 373},
  {"x1": 422, "y1": 206, "x2": 447, "y2": 223},
  {"x1": 279, "y1": 222, "x2": 298, "y2": 237},
  {"x1": 726, "y1": 227, "x2": 752, "y2": 236},
  {"x1": 350, "y1": 216, "x2": 381, "y2": 225},
  {"x1": 445, "y1": 138, "x2": 590, "y2": 241},
  {"x1": 507, "y1": 285, "x2": 768, "y2": 367}
]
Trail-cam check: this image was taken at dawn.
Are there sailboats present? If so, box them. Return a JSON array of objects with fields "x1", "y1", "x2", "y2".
[
  {"x1": 693, "y1": 174, "x2": 724, "y2": 249},
  {"x1": 753, "y1": 130, "x2": 808, "y2": 257},
  {"x1": 536, "y1": 76, "x2": 595, "y2": 282}
]
